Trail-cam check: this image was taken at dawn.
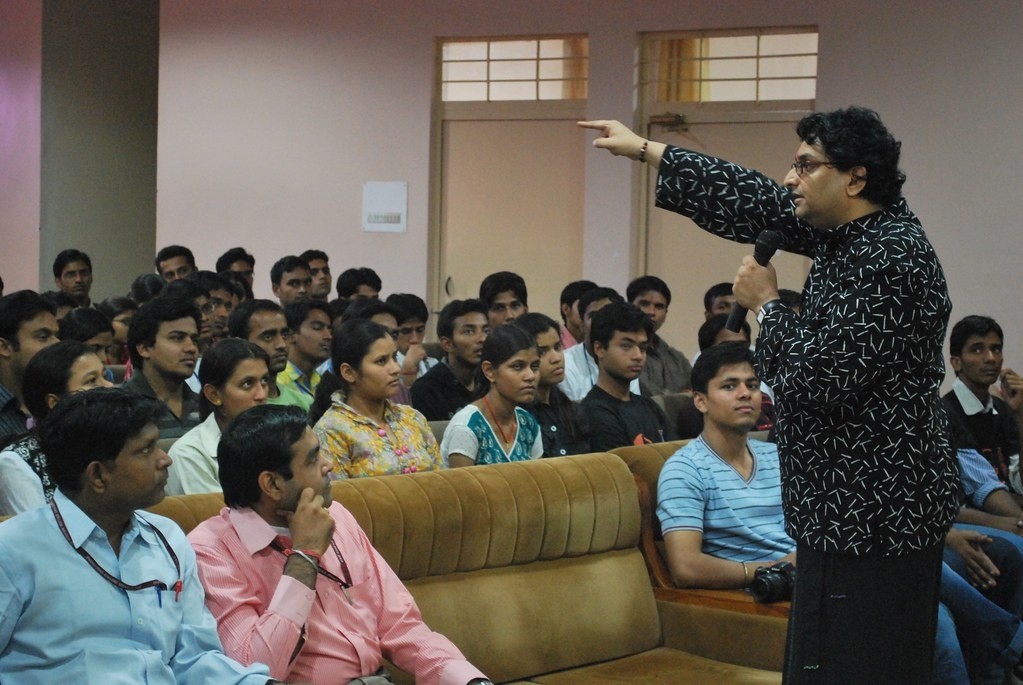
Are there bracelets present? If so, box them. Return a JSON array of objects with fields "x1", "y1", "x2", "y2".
[
  {"x1": 640, "y1": 140, "x2": 650, "y2": 163},
  {"x1": 291, "y1": 549, "x2": 319, "y2": 573},
  {"x1": 741, "y1": 561, "x2": 747, "y2": 589},
  {"x1": 401, "y1": 370, "x2": 417, "y2": 376},
  {"x1": 281, "y1": 548, "x2": 321, "y2": 562}
]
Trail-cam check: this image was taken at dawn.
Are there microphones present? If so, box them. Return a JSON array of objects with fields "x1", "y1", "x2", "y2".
[{"x1": 725, "y1": 230, "x2": 779, "y2": 333}]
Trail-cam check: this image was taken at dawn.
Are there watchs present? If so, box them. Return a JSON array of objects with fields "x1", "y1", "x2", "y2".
[
  {"x1": 477, "y1": 681, "x2": 493, "y2": 685},
  {"x1": 756, "y1": 298, "x2": 787, "y2": 326}
]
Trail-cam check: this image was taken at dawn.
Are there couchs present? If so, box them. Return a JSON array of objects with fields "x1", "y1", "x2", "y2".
[{"x1": 141, "y1": 428, "x2": 791, "y2": 684}]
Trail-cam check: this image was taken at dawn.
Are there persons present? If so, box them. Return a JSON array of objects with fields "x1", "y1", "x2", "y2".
[
  {"x1": 578, "y1": 105, "x2": 959, "y2": 684},
  {"x1": 0, "y1": 245, "x2": 1022, "y2": 685}
]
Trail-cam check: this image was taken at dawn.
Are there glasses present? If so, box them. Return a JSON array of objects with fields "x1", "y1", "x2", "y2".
[
  {"x1": 197, "y1": 301, "x2": 215, "y2": 317},
  {"x1": 791, "y1": 160, "x2": 846, "y2": 175},
  {"x1": 109, "y1": 318, "x2": 132, "y2": 325}
]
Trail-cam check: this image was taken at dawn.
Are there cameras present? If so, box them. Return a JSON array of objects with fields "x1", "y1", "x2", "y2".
[{"x1": 750, "y1": 561, "x2": 796, "y2": 603}]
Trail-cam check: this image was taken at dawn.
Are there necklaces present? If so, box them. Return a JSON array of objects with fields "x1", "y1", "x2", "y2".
[{"x1": 483, "y1": 396, "x2": 515, "y2": 449}]
